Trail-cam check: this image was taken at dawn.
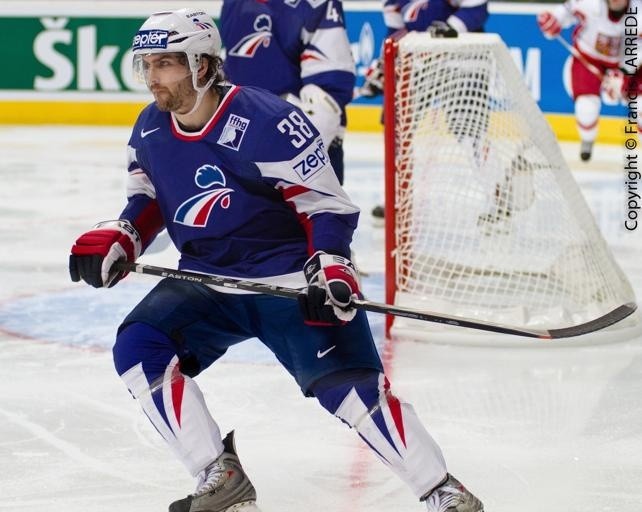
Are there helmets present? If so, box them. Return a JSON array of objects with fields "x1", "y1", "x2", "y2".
[{"x1": 130, "y1": 10, "x2": 222, "y2": 92}]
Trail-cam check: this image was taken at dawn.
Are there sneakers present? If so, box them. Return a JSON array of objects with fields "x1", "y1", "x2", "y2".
[
  {"x1": 425, "y1": 475, "x2": 485, "y2": 512},
  {"x1": 581, "y1": 141, "x2": 592, "y2": 160},
  {"x1": 480, "y1": 207, "x2": 510, "y2": 222},
  {"x1": 170, "y1": 430, "x2": 257, "y2": 512},
  {"x1": 372, "y1": 203, "x2": 403, "y2": 217}
]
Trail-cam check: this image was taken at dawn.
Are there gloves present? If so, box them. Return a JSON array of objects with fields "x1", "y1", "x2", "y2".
[
  {"x1": 302, "y1": 251, "x2": 361, "y2": 330},
  {"x1": 538, "y1": 13, "x2": 560, "y2": 42},
  {"x1": 600, "y1": 67, "x2": 625, "y2": 107},
  {"x1": 68, "y1": 217, "x2": 142, "y2": 289}
]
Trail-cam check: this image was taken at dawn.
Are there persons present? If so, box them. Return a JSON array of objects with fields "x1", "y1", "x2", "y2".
[
  {"x1": 534, "y1": 1, "x2": 642, "y2": 163},
  {"x1": 66, "y1": 6, "x2": 488, "y2": 512},
  {"x1": 370, "y1": 0, "x2": 490, "y2": 218},
  {"x1": 217, "y1": 1, "x2": 357, "y2": 187}
]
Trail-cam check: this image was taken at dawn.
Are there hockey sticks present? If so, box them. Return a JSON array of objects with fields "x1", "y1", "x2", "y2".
[{"x1": 112, "y1": 262, "x2": 638, "y2": 339}]
